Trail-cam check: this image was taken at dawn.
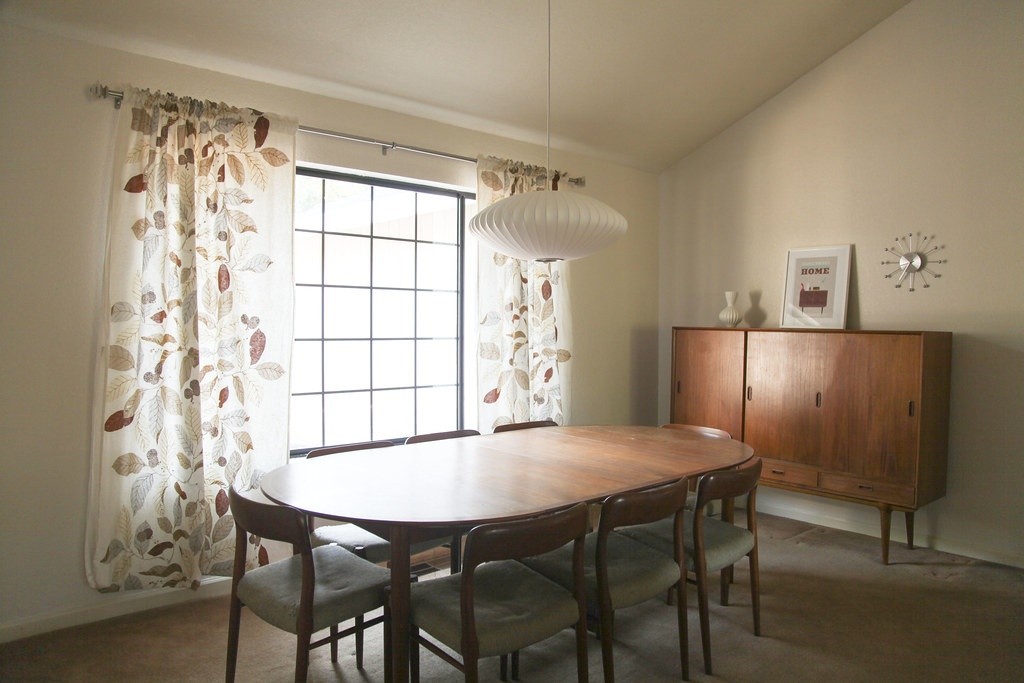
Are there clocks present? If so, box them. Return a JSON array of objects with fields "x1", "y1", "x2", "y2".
[{"x1": 879, "y1": 231, "x2": 944, "y2": 293}]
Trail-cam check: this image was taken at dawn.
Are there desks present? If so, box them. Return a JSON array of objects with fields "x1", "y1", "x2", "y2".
[{"x1": 255, "y1": 427, "x2": 756, "y2": 683}]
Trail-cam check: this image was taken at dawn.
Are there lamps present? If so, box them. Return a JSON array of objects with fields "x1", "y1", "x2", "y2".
[{"x1": 470, "y1": 0, "x2": 628, "y2": 300}]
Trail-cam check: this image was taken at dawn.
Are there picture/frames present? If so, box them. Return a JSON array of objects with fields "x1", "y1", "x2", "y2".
[{"x1": 779, "y1": 245, "x2": 851, "y2": 330}]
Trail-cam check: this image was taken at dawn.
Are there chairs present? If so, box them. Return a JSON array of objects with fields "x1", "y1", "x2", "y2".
[{"x1": 224, "y1": 420, "x2": 763, "y2": 683}]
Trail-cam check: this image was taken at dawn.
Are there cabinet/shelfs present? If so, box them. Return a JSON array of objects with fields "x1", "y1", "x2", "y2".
[
  {"x1": 669, "y1": 324, "x2": 746, "y2": 485},
  {"x1": 747, "y1": 328, "x2": 953, "y2": 564}
]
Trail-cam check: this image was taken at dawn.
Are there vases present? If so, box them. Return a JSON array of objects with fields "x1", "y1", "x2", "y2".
[{"x1": 717, "y1": 290, "x2": 743, "y2": 330}]
